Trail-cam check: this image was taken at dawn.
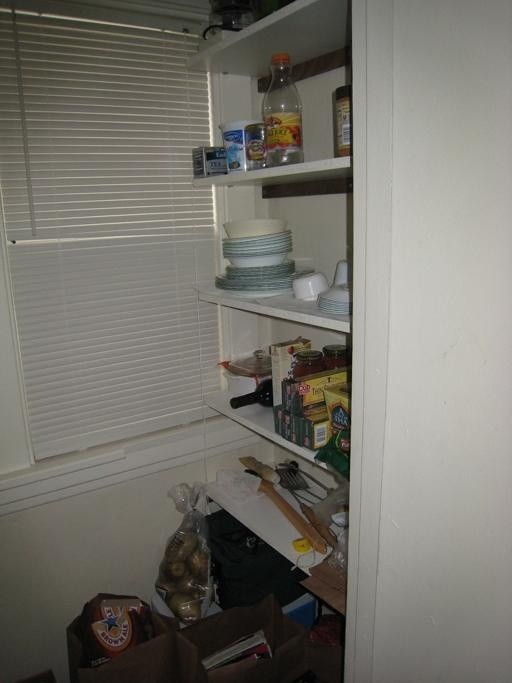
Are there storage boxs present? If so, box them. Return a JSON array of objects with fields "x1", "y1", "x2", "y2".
[
  {"x1": 16, "y1": 667, "x2": 56, "y2": 683},
  {"x1": 66, "y1": 593, "x2": 346, "y2": 683}
]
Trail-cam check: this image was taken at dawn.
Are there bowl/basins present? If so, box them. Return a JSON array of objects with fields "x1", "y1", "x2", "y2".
[
  {"x1": 293, "y1": 273, "x2": 329, "y2": 302},
  {"x1": 220, "y1": 214, "x2": 286, "y2": 240}
]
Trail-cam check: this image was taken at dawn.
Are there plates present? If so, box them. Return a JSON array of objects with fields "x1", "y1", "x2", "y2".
[
  {"x1": 214, "y1": 229, "x2": 312, "y2": 300},
  {"x1": 318, "y1": 289, "x2": 352, "y2": 315}
]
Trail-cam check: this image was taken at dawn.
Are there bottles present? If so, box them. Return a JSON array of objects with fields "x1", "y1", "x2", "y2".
[
  {"x1": 229, "y1": 378, "x2": 274, "y2": 411},
  {"x1": 262, "y1": 49, "x2": 305, "y2": 169}
]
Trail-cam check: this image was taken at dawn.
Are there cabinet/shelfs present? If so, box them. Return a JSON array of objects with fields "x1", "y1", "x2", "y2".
[{"x1": 185, "y1": 0, "x2": 353, "y2": 576}]
